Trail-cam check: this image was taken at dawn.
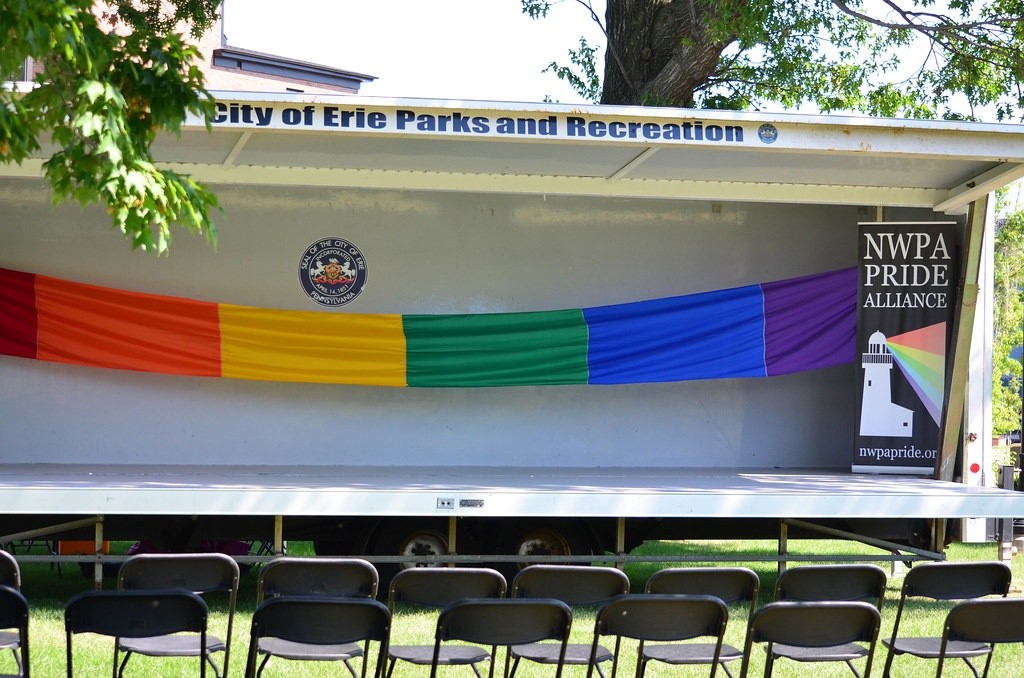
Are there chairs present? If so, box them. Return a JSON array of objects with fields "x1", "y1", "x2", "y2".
[{"x1": 0, "y1": 549, "x2": 1023, "y2": 677}]
